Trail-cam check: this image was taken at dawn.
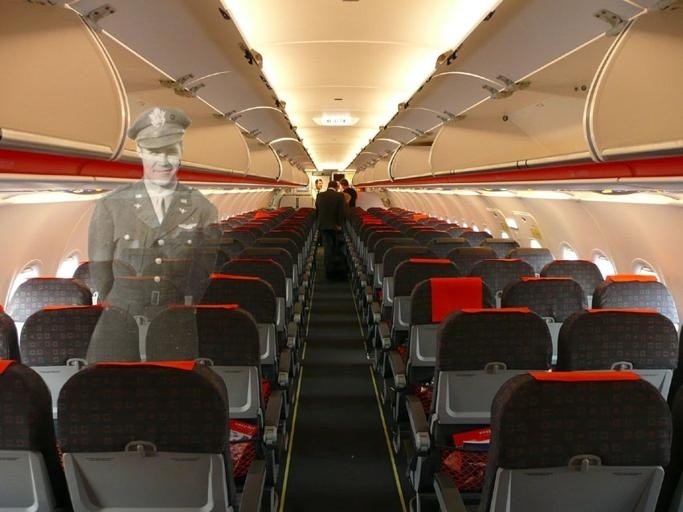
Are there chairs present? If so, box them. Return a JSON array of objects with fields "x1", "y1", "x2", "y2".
[
  {"x1": 0, "y1": 207, "x2": 320, "y2": 512},
  {"x1": 343, "y1": 206, "x2": 681, "y2": 511}
]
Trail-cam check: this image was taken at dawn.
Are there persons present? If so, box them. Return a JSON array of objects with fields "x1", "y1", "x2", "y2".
[
  {"x1": 311, "y1": 178, "x2": 358, "y2": 286},
  {"x1": 79, "y1": 105, "x2": 221, "y2": 365}
]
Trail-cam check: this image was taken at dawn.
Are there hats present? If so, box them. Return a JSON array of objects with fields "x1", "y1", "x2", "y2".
[{"x1": 127, "y1": 106, "x2": 193, "y2": 151}]
